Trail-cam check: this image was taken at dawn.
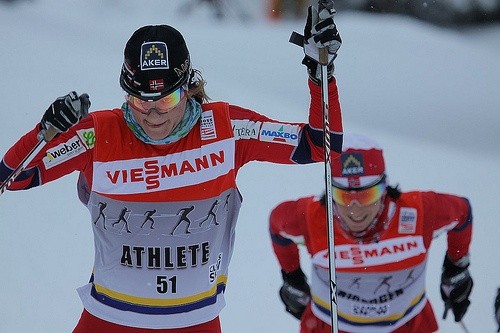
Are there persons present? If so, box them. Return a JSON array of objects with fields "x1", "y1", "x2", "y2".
[
  {"x1": 270, "y1": 149, "x2": 473, "y2": 333},
  {"x1": 0, "y1": 6, "x2": 343, "y2": 333}
]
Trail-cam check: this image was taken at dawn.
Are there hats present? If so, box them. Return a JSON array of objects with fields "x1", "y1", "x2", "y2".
[
  {"x1": 119, "y1": 24, "x2": 192, "y2": 101},
  {"x1": 332, "y1": 147, "x2": 386, "y2": 188}
]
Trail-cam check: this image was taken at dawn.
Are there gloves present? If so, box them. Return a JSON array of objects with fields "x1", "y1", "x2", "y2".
[
  {"x1": 39, "y1": 90, "x2": 91, "y2": 137},
  {"x1": 279, "y1": 267, "x2": 311, "y2": 319},
  {"x1": 290, "y1": 0, "x2": 343, "y2": 84},
  {"x1": 440, "y1": 249, "x2": 473, "y2": 321}
]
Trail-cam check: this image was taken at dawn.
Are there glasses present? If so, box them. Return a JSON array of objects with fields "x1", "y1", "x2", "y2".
[
  {"x1": 122, "y1": 83, "x2": 188, "y2": 115},
  {"x1": 332, "y1": 180, "x2": 387, "y2": 206}
]
